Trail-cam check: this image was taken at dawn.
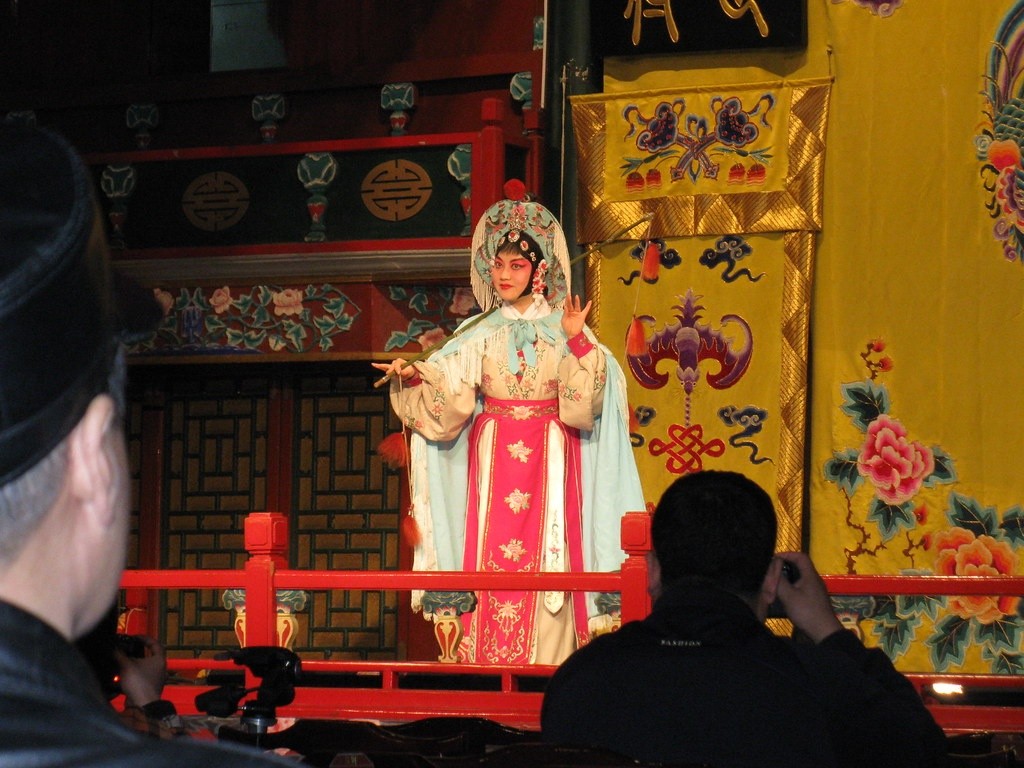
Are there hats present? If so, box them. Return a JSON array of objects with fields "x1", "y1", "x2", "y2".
[{"x1": 1, "y1": 118, "x2": 165, "y2": 489}]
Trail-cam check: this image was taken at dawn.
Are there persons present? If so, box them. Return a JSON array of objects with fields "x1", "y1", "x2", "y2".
[
  {"x1": 539, "y1": 470, "x2": 947, "y2": 768},
  {"x1": 371, "y1": 199, "x2": 649, "y2": 667},
  {"x1": 0, "y1": 109, "x2": 310, "y2": 768}
]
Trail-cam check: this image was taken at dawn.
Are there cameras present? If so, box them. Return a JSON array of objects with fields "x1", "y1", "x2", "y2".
[
  {"x1": 767, "y1": 561, "x2": 798, "y2": 620},
  {"x1": 114, "y1": 632, "x2": 144, "y2": 677}
]
